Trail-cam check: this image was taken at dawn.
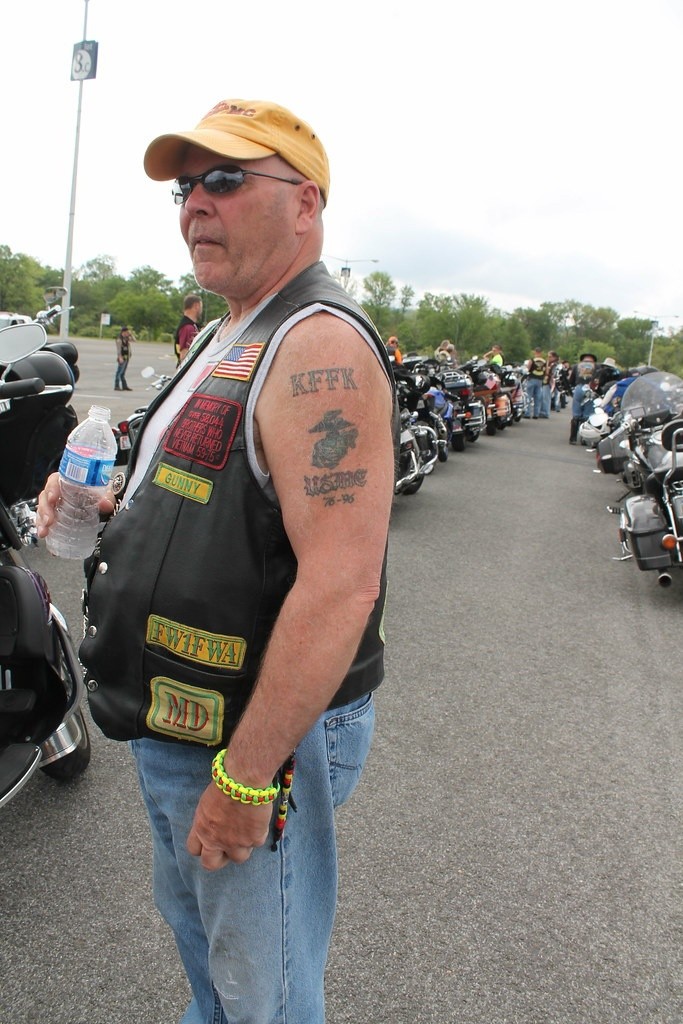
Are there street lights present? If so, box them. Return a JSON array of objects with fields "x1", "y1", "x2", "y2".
[
  {"x1": 328, "y1": 254, "x2": 379, "y2": 294},
  {"x1": 633, "y1": 310, "x2": 679, "y2": 367}
]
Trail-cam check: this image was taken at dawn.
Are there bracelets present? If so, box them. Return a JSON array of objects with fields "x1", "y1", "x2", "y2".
[{"x1": 211, "y1": 749, "x2": 280, "y2": 806}]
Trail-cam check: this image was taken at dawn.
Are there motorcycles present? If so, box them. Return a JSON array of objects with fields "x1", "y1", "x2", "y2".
[
  {"x1": 112, "y1": 346, "x2": 566, "y2": 512},
  {"x1": 0, "y1": 284, "x2": 97, "y2": 809},
  {"x1": 577, "y1": 361, "x2": 683, "y2": 587}
]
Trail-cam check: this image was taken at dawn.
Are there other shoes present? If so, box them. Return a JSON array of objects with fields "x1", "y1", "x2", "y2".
[
  {"x1": 523, "y1": 416, "x2": 538, "y2": 419},
  {"x1": 114, "y1": 386, "x2": 132, "y2": 391}
]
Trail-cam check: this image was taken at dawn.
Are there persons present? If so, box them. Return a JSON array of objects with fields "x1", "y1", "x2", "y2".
[
  {"x1": 36, "y1": 99, "x2": 403, "y2": 1024},
  {"x1": 482, "y1": 344, "x2": 503, "y2": 368},
  {"x1": 524, "y1": 347, "x2": 572, "y2": 419},
  {"x1": 433, "y1": 340, "x2": 455, "y2": 368},
  {"x1": 568, "y1": 354, "x2": 618, "y2": 445}
]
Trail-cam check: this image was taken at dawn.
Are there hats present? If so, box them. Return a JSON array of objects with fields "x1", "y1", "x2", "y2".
[
  {"x1": 580, "y1": 354, "x2": 597, "y2": 363},
  {"x1": 600, "y1": 358, "x2": 617, "y2": 369},
  {"x1": 145, "y1": 97, "x2": 328, "y2": 208}
]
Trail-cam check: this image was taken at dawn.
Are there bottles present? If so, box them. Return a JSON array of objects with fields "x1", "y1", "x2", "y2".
[{"x1": 44, "y1": 405, "x2": 118, "y2": 561}]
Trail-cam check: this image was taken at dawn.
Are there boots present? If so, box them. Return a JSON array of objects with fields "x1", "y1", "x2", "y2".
[{"x1": 569, "y1": 418, "x2": 578, "y2": 444}]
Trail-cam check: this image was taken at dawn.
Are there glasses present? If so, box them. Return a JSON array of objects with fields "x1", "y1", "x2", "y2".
[{"x1": 171, "y1": 165, "x2": 302, "y2": 204}]
